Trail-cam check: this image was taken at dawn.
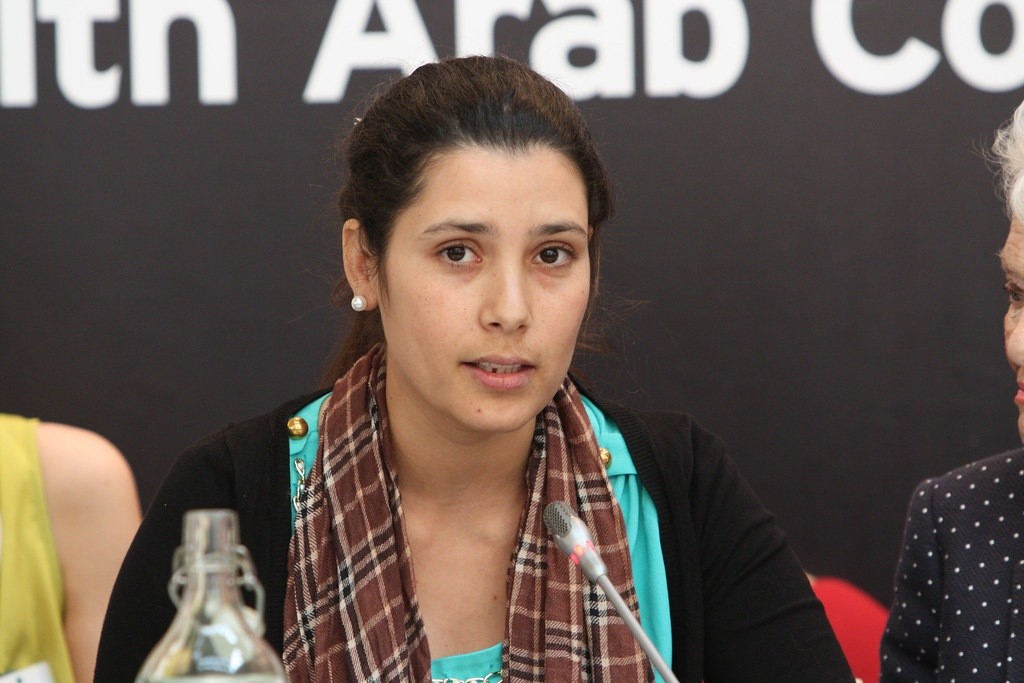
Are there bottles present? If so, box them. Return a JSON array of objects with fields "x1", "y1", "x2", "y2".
[{"x1": 132, "y1": 510, "x2": 290, "y2": 683}]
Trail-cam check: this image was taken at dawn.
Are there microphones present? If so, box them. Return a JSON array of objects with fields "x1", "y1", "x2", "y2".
[{"x1": 543, "y1": 500, "x2": 681, "y2": 683}]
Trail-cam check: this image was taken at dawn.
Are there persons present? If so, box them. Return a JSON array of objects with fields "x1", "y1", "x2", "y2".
[
  {"x1": 92, "y1": 54, "x2": 857, "y2": 683},
  {"x1": 0, "y1": 415, "x2": 142, "y2": 683},
  {"x1": 878, "y1": 101, "x2": 1024, "y2": 683}
]
